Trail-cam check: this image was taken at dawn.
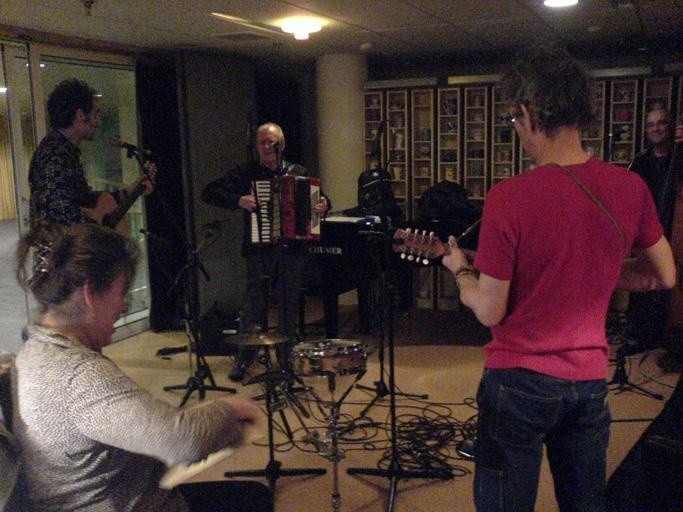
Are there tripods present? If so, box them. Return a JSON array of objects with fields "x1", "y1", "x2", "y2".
[
  {"x1": 224, "y1": 347, "x2": 326, "y2": 512},
  {"x1": 346, "y1": 265, "x2": 452, "y2": 512},
  {"x1": 606, "y1": 291, "x2": 663, "y2": 400},
  {"x1": 251, "y1": 234, "x2": 315, "y2": 418},
  {"x1": 356, "y1": 240, "x2": 428, "y2": 416}
]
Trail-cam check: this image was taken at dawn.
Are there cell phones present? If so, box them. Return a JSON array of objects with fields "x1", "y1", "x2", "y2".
[{"x1": 164, "y1": 256, "x2": 236, "y2": 408}]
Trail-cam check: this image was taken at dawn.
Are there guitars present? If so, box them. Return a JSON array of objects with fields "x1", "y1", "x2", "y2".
[{"x1": 80, "y1": 153, "x2": 156, "y2": 237}]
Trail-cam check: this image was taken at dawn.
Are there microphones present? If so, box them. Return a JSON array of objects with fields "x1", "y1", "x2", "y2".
[
  {"x1": 271, "y1": 139, "x2": 279, "y2": 147},
  {"x1": 604, "y1": 125, "x2": 629, "y2": 140},
  {"x1": 371, "y1": 120, "x2": 384, "y2": 156},
  {"x1": 110, "y1": 138, "x2": 151, "y2": 156}
]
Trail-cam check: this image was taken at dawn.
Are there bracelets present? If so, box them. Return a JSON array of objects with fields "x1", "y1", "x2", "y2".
[{"x1": 454, "y1": 267, "x2": 473, "y2": 279}]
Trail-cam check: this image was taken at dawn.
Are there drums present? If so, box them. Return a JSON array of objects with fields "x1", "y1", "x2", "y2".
[{"x1": 292, "y1": 337, "x2": 366, "y2": 376}]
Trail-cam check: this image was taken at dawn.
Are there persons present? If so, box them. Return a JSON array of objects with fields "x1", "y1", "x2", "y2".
[
  {"x1": 440, "y1": 39, "x2": 680, "y2": 511},
  {"x1": 27, "y1": 78, "x2": 155, "y2": 232},
  {"x1": 202, "y1": 123, "x2": 332, "y2": 380},
  {"x1": 8, "y1": 221, "x2": 273, "y2": 511},
  {"x1": 617, "y1": 104, "x2": 683, "y2": 370}
]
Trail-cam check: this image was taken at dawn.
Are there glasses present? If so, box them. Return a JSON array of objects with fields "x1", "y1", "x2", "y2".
[{"x1": 499, "y1": 105, "x2": 534, "y2": 127}]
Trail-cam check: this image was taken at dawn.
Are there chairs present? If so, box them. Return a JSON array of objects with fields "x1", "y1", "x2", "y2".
[{"x1": 0, "y1": 355, "x2": 31, "y2": 512}]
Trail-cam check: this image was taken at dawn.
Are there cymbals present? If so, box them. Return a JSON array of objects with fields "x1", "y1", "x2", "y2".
[{"x1": 226, "y1": 331, "x2": 290, "y2": 347}]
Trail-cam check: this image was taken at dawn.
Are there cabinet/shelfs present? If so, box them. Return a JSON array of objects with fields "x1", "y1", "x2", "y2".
[{"x1": 359, "y1": 61, "x2": 681, "y2": 327}]
